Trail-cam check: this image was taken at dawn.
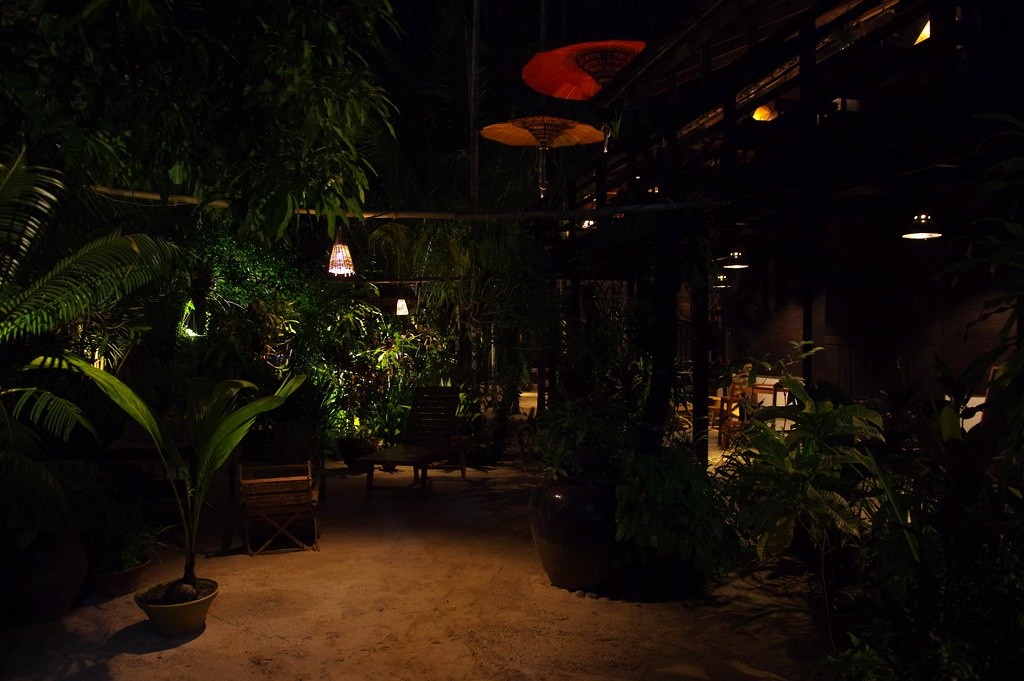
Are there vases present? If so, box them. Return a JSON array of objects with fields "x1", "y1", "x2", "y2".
[{"x1": 528, "y1": 402, "x2": 729, "y2": 601}]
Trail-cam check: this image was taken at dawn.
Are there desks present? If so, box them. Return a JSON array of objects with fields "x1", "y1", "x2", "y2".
[{"x1": 741, "y1": 383, "x2": 799, "y2": 436}]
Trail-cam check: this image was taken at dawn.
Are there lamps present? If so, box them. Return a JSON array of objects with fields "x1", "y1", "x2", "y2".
[
  {"x1": 397, "y1": 286, "x2": 409, "y2": 317},
  {"x1": 326, "y1": 224, "x2": 358, "y2": 278}
]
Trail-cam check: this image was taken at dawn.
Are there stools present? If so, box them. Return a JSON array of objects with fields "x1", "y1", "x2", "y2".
[{"x1": 716, "y1": 396, "x2": 747, "y2": 450}]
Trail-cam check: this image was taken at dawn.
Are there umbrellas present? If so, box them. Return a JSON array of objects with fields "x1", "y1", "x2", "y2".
[
  {"x1": 480, "y1": 117, "x2": 608, "y2": 198},
  {"x1": 523, "y1": 40, "x2": 648, "y2": 152}
]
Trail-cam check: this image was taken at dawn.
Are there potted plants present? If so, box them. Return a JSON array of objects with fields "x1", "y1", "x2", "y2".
[
  {"x1": 1, "y1": 355, "x2": 186, "y2": 625},
  {"x1": 60, "y1": 353, "x2": 308, "y2": 637}
]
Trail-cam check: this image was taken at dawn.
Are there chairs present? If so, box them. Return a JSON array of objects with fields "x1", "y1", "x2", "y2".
[{"x1": 357, "y1": 383, "x2": 473, "y2": 505}]
